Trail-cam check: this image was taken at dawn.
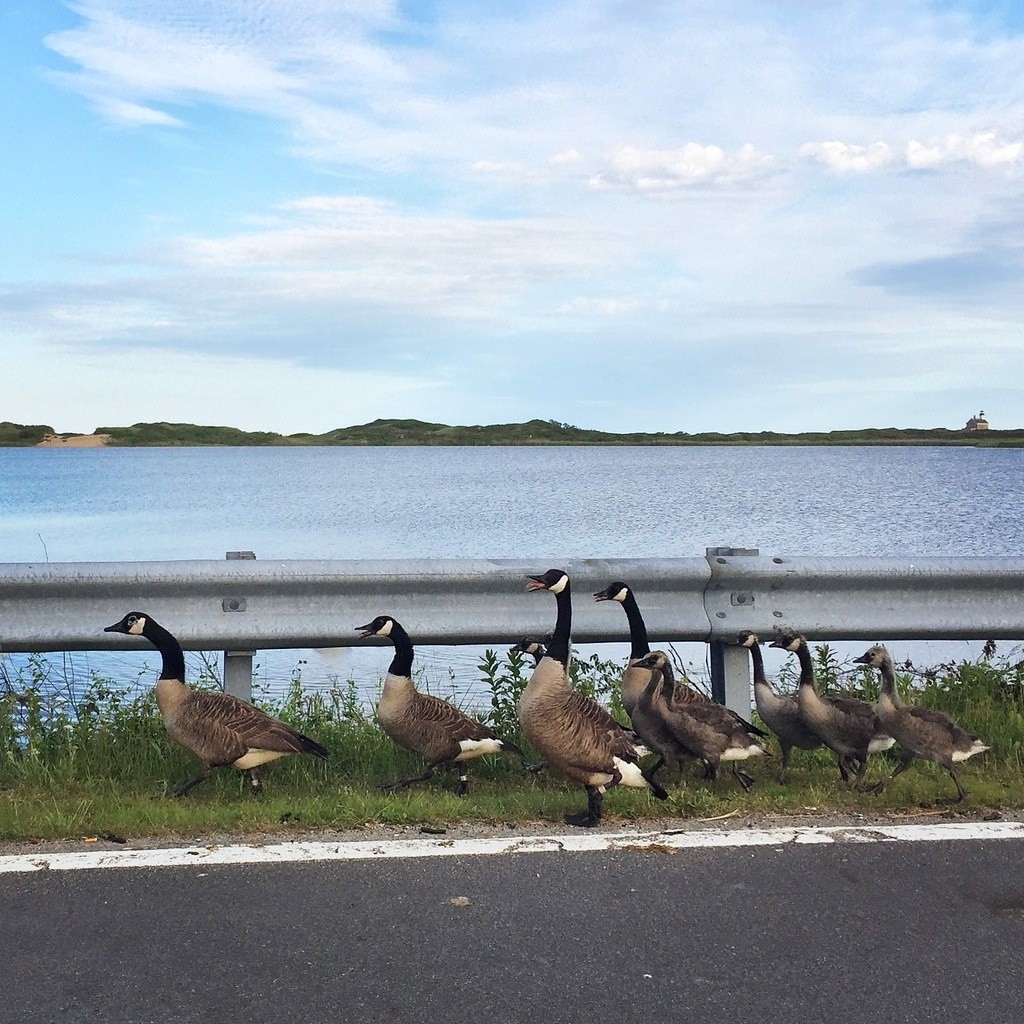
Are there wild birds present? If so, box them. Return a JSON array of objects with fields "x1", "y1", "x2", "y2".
[
  {"x1": 510, "y1": 636, "x2": 654, "y2": 758},
  {"x1": 727, "y1": 630, "x2": 827, "y2": 768},
  {"x1": 853, "y1": 647, "x2": 991, "y2": 803},
  {"x1": 103, "y1": 611, "x2": 329, "y2": 802},
  {"x1": 769, "y1": 632, "x2": 897, "y2": 792},
  {"x1": 353, "y1": 616, "x2": 523, "y2": 798},
  {"x1": 593, "y1": 581, "x2": 774, "y2": 794},
  {"x1": 515, "y1": 569, "x2": 669, "y2": 827}
]
